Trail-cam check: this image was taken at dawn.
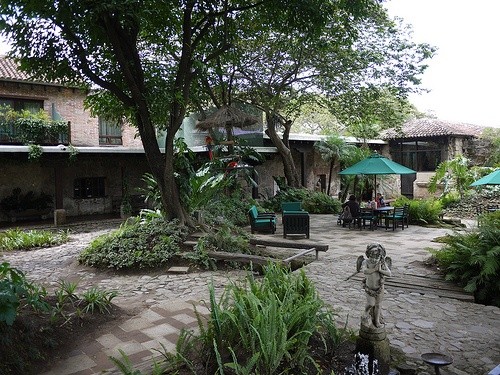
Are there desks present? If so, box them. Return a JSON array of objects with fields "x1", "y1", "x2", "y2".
[{"x1": 360, "y1": 207, "x2": 394, "y2": 227}]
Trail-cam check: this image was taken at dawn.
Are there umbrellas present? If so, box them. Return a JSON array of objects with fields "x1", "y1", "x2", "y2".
[
  {"x1": 338, "y1": 150, "x2": 417, "y2": 202},
  {"x1": 469, "y1": 169, "x2": 500, "y2": 186}
]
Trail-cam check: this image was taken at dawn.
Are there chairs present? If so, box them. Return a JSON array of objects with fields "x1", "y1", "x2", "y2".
[
  {"x1": 282, "y1": 202, "x2": 304, "y2": 224},
  {"x1": 342, "y1": 202, "x2": 411, "y2": 231},
  {"x1": 248, "y1": 205, "x2": 278, "y2": 234},
  {"x1": 283, "y1": 211, "x2": 310, "y2": 239},
  {"x1": 477, "y1": 202, "x2": 498, "y2": 227}
]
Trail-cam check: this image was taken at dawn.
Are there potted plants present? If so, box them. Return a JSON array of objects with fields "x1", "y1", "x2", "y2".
[{"x1": 119, "y1": 178, "x2": 133, "y2": 219}]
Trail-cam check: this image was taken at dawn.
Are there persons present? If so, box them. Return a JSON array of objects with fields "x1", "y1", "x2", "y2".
[
  {"x1": 377, "y1": 193, "x2": 386, "y2": 207},
  {"x1": 363, "y1": 242, "x2": 391, "y2": 328},
  {"x1": 342, "y1": 195, "x2": 361, "y2": 217}
]
